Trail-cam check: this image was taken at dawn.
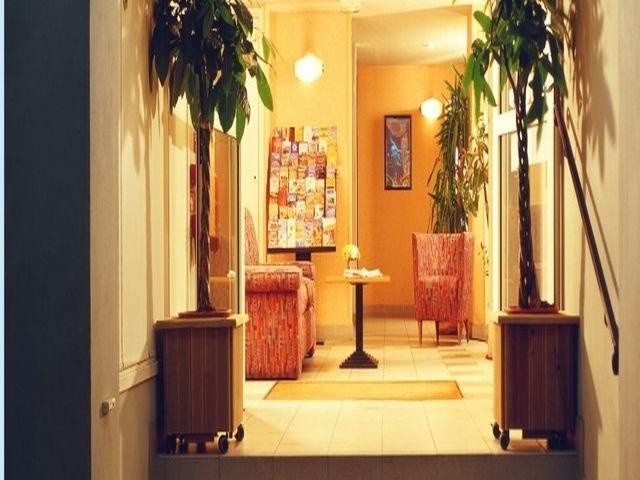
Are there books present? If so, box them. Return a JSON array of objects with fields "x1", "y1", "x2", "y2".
[{"x1": 266, "y1": 124, "x2": 339, "y2": 250}]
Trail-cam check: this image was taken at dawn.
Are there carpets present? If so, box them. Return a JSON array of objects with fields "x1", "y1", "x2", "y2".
[{"x1": 262, "y1": 380, "x2": 468, "y2": 401}]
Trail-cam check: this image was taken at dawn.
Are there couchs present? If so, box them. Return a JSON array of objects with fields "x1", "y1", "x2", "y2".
[{"x1": 245, "y1": 202, "x2": 319, "y2": 381}]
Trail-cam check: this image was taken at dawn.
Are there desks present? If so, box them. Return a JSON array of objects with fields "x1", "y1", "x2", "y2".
[{"x1": 324, "y1": 275, "x2": 393, "y2": 370}]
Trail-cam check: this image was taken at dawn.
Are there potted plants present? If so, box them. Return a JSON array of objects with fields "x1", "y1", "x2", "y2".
[
  {"x1": 459, "y1": 0, "x2": 582, "y2": 454},
  {"x1": 146, "y1": 0, "x2": 282, "y2": 458}
]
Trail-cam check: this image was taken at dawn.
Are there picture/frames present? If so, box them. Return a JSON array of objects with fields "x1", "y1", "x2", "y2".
[{"x1": 382, "y1": 114, "x2": 414, "y2": 192}]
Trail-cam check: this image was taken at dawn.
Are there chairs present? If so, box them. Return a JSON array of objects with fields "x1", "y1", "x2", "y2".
[{"x1": 411, "y1": 230, "x2": 476, "y2": 348}]
